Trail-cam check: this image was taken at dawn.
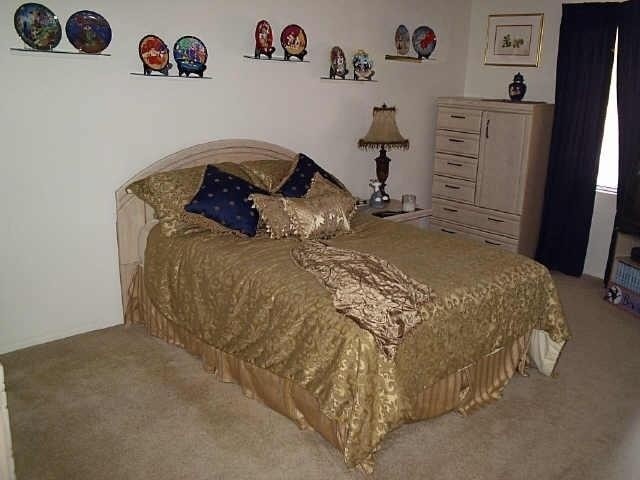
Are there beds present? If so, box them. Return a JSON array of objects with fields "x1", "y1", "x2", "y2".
[{"x1": 114, "y1": 138, "x2": 572, "y2": 476}]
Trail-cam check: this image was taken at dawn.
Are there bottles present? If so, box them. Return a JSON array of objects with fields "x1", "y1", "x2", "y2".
[{"x1": 508, "y1": 73, "x2": 528, "y2": 102}]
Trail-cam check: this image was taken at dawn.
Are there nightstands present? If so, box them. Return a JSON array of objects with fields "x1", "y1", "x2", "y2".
[{"x1": 358, "y1": 199, "x2": 427, "y2": 230}]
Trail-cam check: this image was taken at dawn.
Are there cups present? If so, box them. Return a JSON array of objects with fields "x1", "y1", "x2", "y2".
[{"x1": 400, "y1": 194, "x2": 416, "y2": 213}]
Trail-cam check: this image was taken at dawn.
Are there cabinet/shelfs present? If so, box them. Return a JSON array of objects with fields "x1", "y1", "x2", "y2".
[{"x1": 428, "y1": 97, "x2": 556, "y2": 261}]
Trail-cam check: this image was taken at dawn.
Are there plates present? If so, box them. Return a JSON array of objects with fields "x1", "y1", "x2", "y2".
[
  {"x1": 138, "y1": 34, "x2": 208, "y2": 72},
  {"x1": 328, "y1": 46, "x2": 375, "y2": 77},
  {"x1": 394, "y1": 24, "x2": 437, "y2": 59},
  {"x1": 254, "y1": 19, "x2": 308, "y2": 55},
  {"x1": 14, "y1": 2, "x2": 113, "y2": 54}
]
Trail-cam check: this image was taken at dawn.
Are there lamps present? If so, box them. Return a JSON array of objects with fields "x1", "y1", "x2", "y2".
[{"x1": 357, "y1": 102, "x2": 410, "y2": 201}]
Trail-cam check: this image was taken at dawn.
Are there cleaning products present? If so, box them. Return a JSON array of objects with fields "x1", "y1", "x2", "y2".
[{"x1": 368, "y1": 180, "x2": 385, "y2": 209}]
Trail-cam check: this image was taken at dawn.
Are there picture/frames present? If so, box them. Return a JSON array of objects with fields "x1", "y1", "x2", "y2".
[{"x1": 483, "y1": 13, "x2": 544, "y2": 68}]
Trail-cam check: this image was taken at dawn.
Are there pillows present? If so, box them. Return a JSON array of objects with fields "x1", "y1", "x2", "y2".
[{"x1": 125, "y1": 153, "x2": 359, "y2": 242}]
